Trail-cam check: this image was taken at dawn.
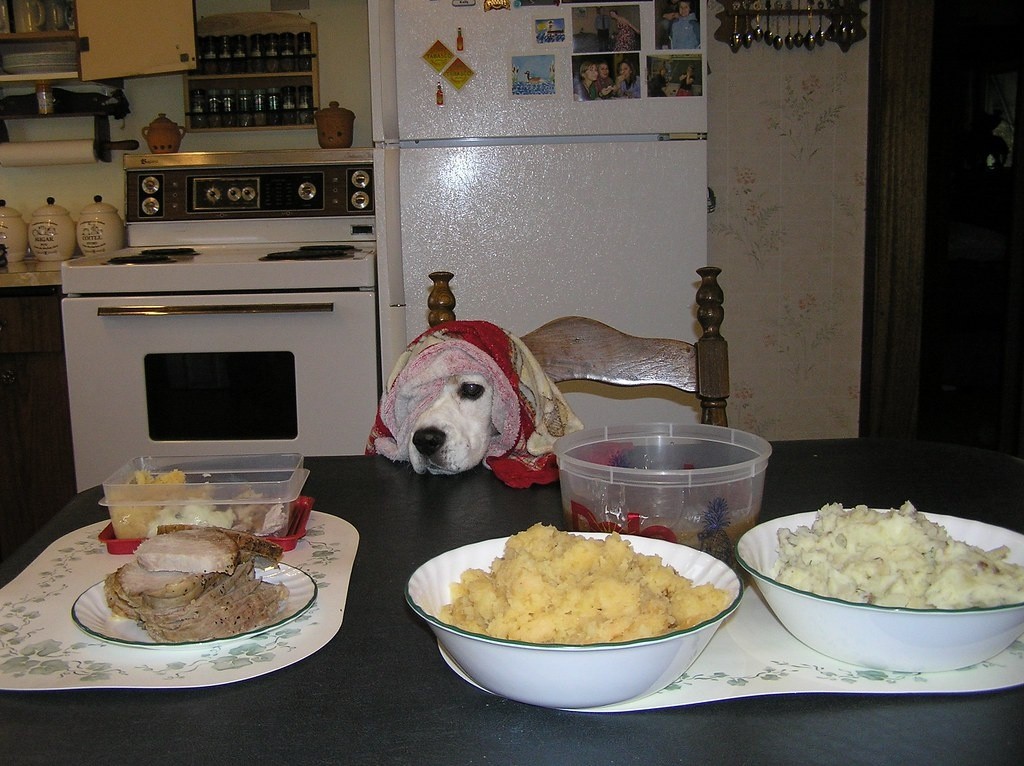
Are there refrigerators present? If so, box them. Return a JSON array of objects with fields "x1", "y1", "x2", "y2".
[{"x1": 389, "y1": 2, "x2": 712, "y2": 456}]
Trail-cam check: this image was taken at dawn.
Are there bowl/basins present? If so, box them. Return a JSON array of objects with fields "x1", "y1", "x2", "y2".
[
  {"x1": 737, "y1": 508, "x2": 1024, "y2": 672},
  {"x1": 403, "y1": 531, "x2": 743, "y2": 710},
  {"x1": 549, "y1": 422, "x2": 772, "y2": 595}
]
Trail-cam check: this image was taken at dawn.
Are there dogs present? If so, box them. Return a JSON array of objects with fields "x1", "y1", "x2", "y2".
[{"x1": 408, "y1": 373, "x2": 491, "y2": 475}]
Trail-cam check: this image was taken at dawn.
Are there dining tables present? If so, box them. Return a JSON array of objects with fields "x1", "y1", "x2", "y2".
[{"x1": 0, "y1": 438, "x2": 1024, "y2": 766}]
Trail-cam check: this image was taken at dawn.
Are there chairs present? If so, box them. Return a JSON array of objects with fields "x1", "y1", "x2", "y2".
[{"x1": 427, "y1": 265, "x2": 730, "y2": 429}]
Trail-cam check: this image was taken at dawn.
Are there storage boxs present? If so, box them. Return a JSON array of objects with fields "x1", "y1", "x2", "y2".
[{"x1": 96, "y1": 454, "x2": 311, "y2": 537}]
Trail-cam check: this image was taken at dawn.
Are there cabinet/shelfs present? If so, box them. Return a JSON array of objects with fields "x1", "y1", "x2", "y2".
[{"x1": 181, "y1": 9, "x2": 323, "y2": 135}]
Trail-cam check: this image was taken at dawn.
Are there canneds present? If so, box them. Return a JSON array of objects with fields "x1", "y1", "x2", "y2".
[
  {"x1": 35, "y1": 80, "x2": 54, "y2": 115},
  {"x1": 191, "y1": 31, "x2": 315, "y2": 129}
]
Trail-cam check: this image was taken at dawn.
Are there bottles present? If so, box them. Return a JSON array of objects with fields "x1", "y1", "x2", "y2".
[
  {"x1": 0, "y1": 199, "x2": 28, "y2": 262},
  {"x1": 60, "y1": 143, "x2": 382, "y2": 296},
  {"x1": 187, "y1": 31, "x2": 314, "y2": 127},
  {"x1": 27, "y1": 198, "x2": 76, "y2": 262},
  {"x1": 74, "y1": 194, "x2": 128, "y2": 256}
]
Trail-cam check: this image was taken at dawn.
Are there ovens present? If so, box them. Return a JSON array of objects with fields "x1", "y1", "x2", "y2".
[{"x1": 60, "y1": 292, "x2": 379, "y2": 504}]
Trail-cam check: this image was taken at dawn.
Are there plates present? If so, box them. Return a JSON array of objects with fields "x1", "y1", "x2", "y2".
[
  {"x1": 69, "y1": 562, "x2": 319, "y2": 648},
  {"x1": 1, "y1": 51, "x2": 80, "y2": 74}
]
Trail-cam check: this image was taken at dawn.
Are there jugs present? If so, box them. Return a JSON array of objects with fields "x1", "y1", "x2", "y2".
[{"x1": 0, "y1": 0, "x2": 66, "y2": 36}]
